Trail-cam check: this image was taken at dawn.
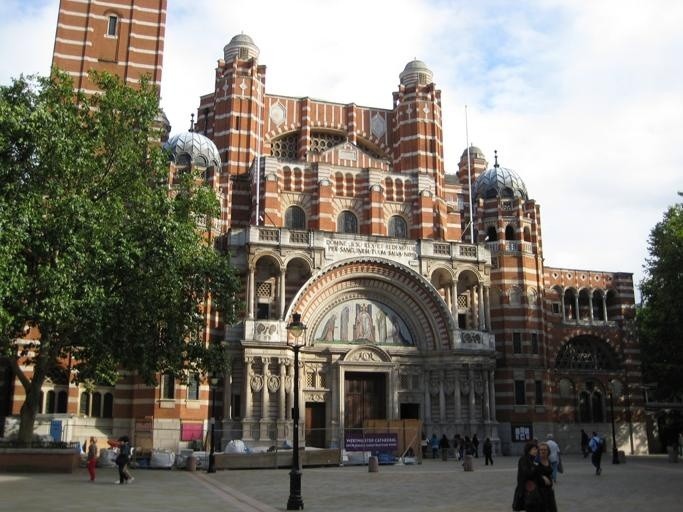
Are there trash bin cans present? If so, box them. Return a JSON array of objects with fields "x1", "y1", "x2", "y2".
[
  {"x1": 617, "y1": 451, "x2": 624, "y2": 462},
  {"x1": 464, "y1": 454, "x2": 473, "y2": 472},
  {"x1": 368, "y1": 457, "x2": 378, "y2": 473},
  {"x1": 187, "y1": 456, "x2": 197, "y2": 472}
]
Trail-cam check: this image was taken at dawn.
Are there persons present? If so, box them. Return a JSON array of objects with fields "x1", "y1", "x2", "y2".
[
  {"x1": 535, "y1": 441, "x2": 557, "y2": 511},
  {"x1": 356, "y1": 303, "x2": 373, "y2": 342},
  {"x1": 316, "y1": 314, "x2": 338, "y2": 342},
  {"x1": 587, "y1": 430, "x2": 604, "y2": 476},
  {"x1": 481, "y1": 436, "x2": 494, "y2": 466},
  {"x1": 421, "y1": 431, "x2": 479, "y2": 466},
  {"x1": 390, "y1": 315, "x2": 408, "y2": 344},
  {"x1": 579, "y1": 428, "x2": 589, "y2": 457},
  {"x1": 511, "y1": 438, "x2": 545, "y2": 511},
  {"x1": 85, "y1": 435, "x2": 99, "y2": 483},
  {"x1": 105, "y1": 434, "x2": 134, "y2": 485},
  {"x1": 543, "y1": 432, "x2": 561, "y2": 482}
]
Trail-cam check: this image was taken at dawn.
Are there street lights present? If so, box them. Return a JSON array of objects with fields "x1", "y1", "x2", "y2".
[
  {"x1": 204, "y1": 371, "x2": 220, "y2": 474},
  {"x1": 282, "y1": 309, "x2": 309, "y2": 512},
  {"x1": 605, "y1": 376, "x2": 618, "y2": 465}
]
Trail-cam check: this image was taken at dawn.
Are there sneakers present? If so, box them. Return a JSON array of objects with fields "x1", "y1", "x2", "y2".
[{"x1": 116, "y1": 477, "x2": 133, "y2": 484}]
